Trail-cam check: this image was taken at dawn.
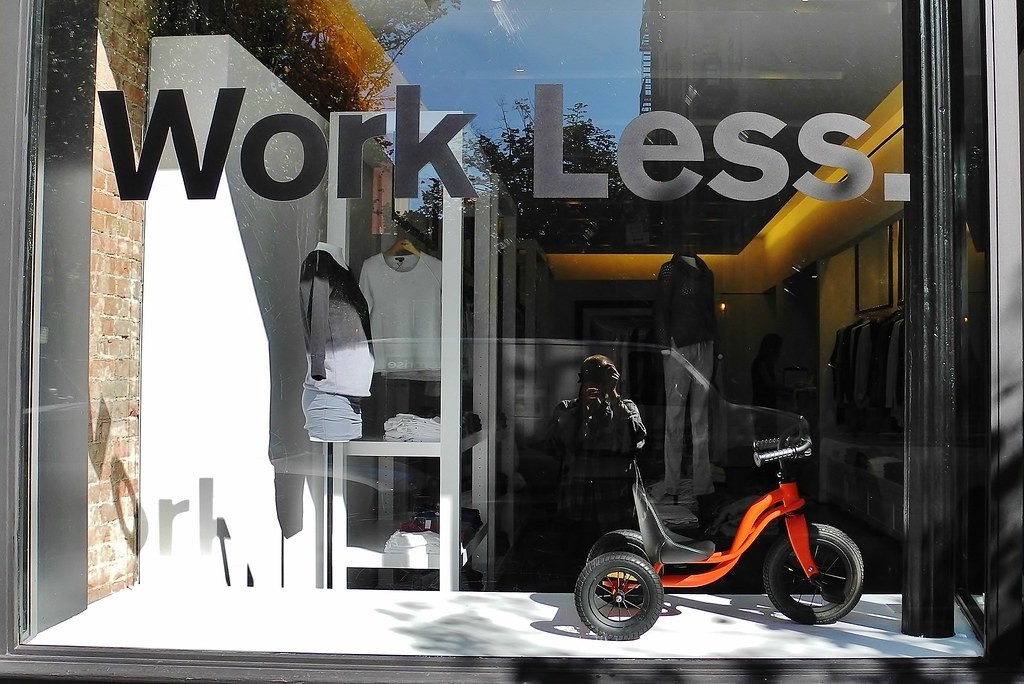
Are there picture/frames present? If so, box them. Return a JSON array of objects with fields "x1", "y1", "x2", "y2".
[{"x1": 574, "y1": 300, "x2": 667, "y2": 441}]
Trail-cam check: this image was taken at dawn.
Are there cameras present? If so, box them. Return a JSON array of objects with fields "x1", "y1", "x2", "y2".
[{"x1": 586, "y1": 365, "x2": 608, "y2": 384}]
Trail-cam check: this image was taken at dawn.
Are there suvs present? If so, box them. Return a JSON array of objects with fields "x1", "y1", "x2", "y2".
[{"x1": 359, "y1": 336, "x2": 811, "y2": 490}]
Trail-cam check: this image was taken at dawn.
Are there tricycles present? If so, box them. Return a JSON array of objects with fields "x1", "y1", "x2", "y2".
[{"x1": 573, "y1": 434, "x2": 867, "y2": 643}]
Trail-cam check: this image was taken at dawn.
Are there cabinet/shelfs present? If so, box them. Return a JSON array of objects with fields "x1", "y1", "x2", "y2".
[
  {"x1": 818, "y1": 210, "x2": 906, "y2": 540},
  {"x1": 330, "y1": 110, "x2": 558, "y2": 593}
]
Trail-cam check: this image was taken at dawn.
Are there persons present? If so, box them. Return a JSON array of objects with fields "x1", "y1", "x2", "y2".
[
  {"x1": 537, "y1": 355, "x2": 648, "y2": 592},
  {"x1": 298, "y1": 242, "x2": 375, "y2": 443},
  {"x1": 656, "y1": 246, "x2": 719, "y2": 495},
  {"x1": 751, "y1": 332, "x2": 795, "y2": 481}
]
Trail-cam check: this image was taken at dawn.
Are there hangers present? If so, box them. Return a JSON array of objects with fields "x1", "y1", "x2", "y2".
[
  {"x1": 860, "y1": 299, "x2": 906, "y2": 323},
  {"x1": 382, "y1": 218, "x2": 419, "y2": 257}
]
